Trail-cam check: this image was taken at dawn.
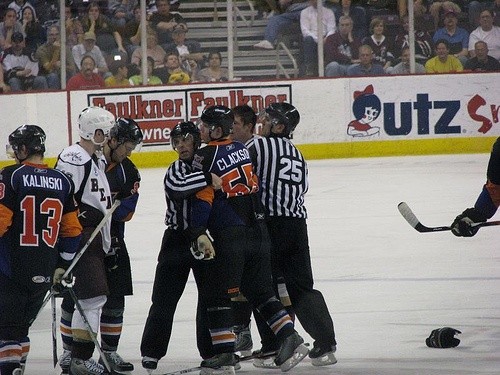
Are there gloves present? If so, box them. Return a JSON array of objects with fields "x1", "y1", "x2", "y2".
[
  {"x1": 189, "y1": 233, "x2": 215, "y2": 262},
  {"x1": 425, "y1": 327, "x2": 462, "y2": 348},
  {"x1": 450, "y1": 208, "x2": 487, "y2": 237},
  {"x1": 52, "y1": 268, "x2": 77, "y2": 296},
  {"x1": 104, "y1": 232, "x2": 121, "y2": 268}
]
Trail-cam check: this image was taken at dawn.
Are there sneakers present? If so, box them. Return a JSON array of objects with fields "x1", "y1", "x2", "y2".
[
  {"x1": 141, "y1": 356, "x2": 158, "y2": 375},
  {"x1": 97, "y1": 350, "x2": 134, "y2": 375},
  {"x1": 58, "y1": 349, "x2": 109, "y2": 375},
  {"x1": 200, "y1": 330, "x2": 338, "y2": 375}
]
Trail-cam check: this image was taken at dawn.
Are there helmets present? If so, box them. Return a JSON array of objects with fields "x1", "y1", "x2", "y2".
[
  {"x1": 263, "y1": 102, "x2": 301, "y2": 131},
  {"x1": 9, "y1": 124, "x2": 46, "y2": 154},
  {"x1": 168, "y1": 122, "x2": 201, "y2": 150},
  {"x1": 112, "y1": 117, "x2": 142, "y2": 145},
  {"x1": 201, "y1": 104, "x2": 235, "y2": 128},
  {"x1": 77, "y1": 106, "x2": 116, "y2": 140}
]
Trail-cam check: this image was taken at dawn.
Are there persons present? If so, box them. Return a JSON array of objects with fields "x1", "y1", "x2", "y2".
[
  {"x1": 0, "y1": 0, "x2": 199, "y2": 56},
  {"x1": 345, "y1": 45, "x2": 384, "y2": 76},
  {"x1": 58, "y1": 117, "x2": 142, "y2": 375},
  {"x1": 450, "y1": 136, "x2": 500, "y2": 237},
  {"x1": 66, "y1": 55, "x2": 105, "y2": 90},
  {"x1": 36, "y1": 25, "x2": 77, "y2": 88},
  {"x1": 191, "y1": 103, "x2": 338, "y2": 375},
  {"x1": 424, "y1": 39, "x2": 463, "y2": 73},
  {"x1": 386, "y1": 46, "x2": 426, "y2": 75},
  {"x1": 198, "y1": 49, "x2": 229, "y2": 82},
  {"x1": 1, "y1": 32, "x2": 48, "y2": 92},
  {"x1": 433, "y1": 7, "x2": 469, "y2": 69},
  {"x1": 0, "y1": 125, "x2": 83, "y2": 375},
  {"x1": 160, "y1": 55, "x2": 197, "y2": 83},
  {"x1": 54, "y1": 106, "x2": 118, "y2": 375},
  {"x1": 129, "y1": 56, "x2": 163, "y2": 86},
  {"x1": 105, "y1": 60, "x2": 132, "y2": 87},
  {"x1": 323, "y1": 16, "x2": 362, "y2": 76},
  {"x1": 72, "y1": 32, "x2": 108, "y2": 73},
  {"x1": 138, "y1": 121, "x2": 223, "y2": 375},
  {"x1": 253, "y1": 0, "x2": 500, "y2": 76}
]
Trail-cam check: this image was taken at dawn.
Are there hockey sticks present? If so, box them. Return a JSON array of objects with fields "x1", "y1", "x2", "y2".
[
  {"x1": 398, "y1": 202, "x2": 500, "y2": 233},
  {"x1": 29, "y1": 199, "x2": 121, "y2": 326},
  {"x1": 67, "y1": 286, "x2": 126, "y2": 375},
  {"x1": 161, "y1": 342, "x2": 311, "y2": 375},
  {"x1": 51, "y1": 292, "x2": 60, "y2": 368}
]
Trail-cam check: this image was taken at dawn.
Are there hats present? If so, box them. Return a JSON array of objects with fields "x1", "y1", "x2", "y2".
[
  {"x1": 443, "y1": 6, "x2": 457, "y2": 17},
  {"x1": 83, "y1": 32, "x2": 96, "y2": 41},
  {"x1": 12, "y1": 32, "x2": 24, "y2": 41},
  {"x1": 171, "y1": 25, "x2": 188, "y2": 33}
]
